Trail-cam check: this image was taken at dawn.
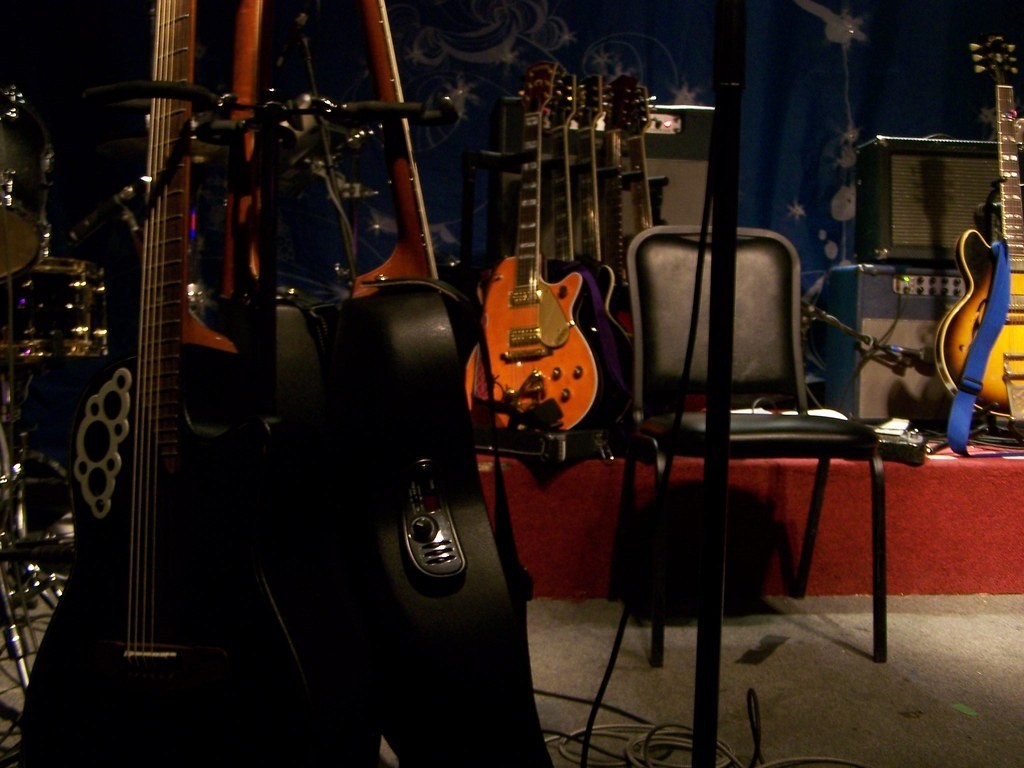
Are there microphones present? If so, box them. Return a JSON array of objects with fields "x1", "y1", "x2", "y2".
[{"x1": 888, "y1": 344, "x2": 936, "y2": 366}]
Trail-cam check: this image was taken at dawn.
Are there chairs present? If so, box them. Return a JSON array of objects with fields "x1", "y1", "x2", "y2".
[{"x1": 609, "y1": 223, "x2": 889, "y2": 669}]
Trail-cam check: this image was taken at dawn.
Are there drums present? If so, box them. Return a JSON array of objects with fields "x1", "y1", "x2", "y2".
[
  {"x1": 21, "y1": 259, "x2": 106, "y2": 358},
  {"x1": 0, "y1": 85, "x2": 39, "y2": 277}
]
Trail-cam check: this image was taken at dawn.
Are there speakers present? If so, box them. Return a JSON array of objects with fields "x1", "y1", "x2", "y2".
[
  {"x1": 851, "y1": 136, "x2": 1003, "y2": 267},
  {"x1": 828, "y1": 264, "x2": 973, "y2": 427}
]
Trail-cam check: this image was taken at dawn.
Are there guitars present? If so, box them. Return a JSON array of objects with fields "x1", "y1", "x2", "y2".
[
  {"x1": 933, "y1": 34, "x2": 1024, "y2": 423},
  {"x1": 462, "y1": 59, "x2": 655, "y2": 445},
  {"x1": 359, "y1": 1, "x2": 561, "y2": 768},
  {"x1": 17, "y1": 0, "x2": 384, "y2": 767}
]
